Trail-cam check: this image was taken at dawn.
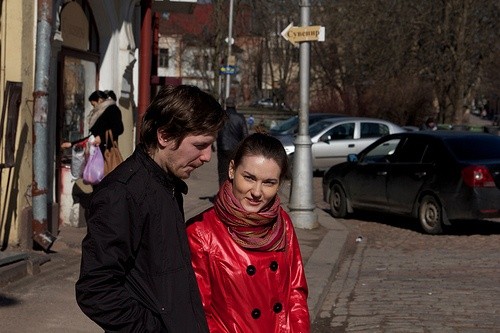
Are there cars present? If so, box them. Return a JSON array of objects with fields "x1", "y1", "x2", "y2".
[
  {"x1": 320, "y1": 130, "x2": 500, "y2": 235},
  {"x1": 400, "y1": 123, "x2": 500, "y2": 134},
  {"x1": 264, "y1": 112, "x2": 421, "y2": 178}
]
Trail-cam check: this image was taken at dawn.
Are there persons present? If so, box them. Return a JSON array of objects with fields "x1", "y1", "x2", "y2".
[
  {"x1": 185, "y1": 133, "x2": 312, "y2": 333},
  {"x1": 211, "y1": 97, "x2": 248, "y2": 188},
  {"x1": 75, "y1": 85, "x2": 225, "y2": 333},
  {"x1": 61, "y1": 89, "x2": 123, "y2": 187}
]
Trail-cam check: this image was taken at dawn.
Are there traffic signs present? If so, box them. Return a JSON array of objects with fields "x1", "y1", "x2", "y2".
[{"x1": 281, "y1": 22, "x2": 324, "y2": 49}]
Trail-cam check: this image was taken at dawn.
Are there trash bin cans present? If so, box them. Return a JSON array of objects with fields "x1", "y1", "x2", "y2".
[
  {"x1": 437, "y1": 123, "x2": 449, "y2": 131},
  {"x1": 451, "y1": 125, "x2": 466, "y2": 131},
  {"x1": 481, "y1": 126, "x2": 498, "y2": 131},
  {"x1": 467, "y1": 126, "x2": 484, "y2": 132}
]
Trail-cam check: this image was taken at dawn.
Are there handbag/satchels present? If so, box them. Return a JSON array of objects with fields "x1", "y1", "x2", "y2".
[
  {"x1": 83, "y1": 145, "x2": 103, "y2": 184},
  {"x1": 104, "y1": 130, "x2": 124, "y2": 176},
  {"x1": 71, "y1": 144, "x2": 85, "y2": 180}
]
